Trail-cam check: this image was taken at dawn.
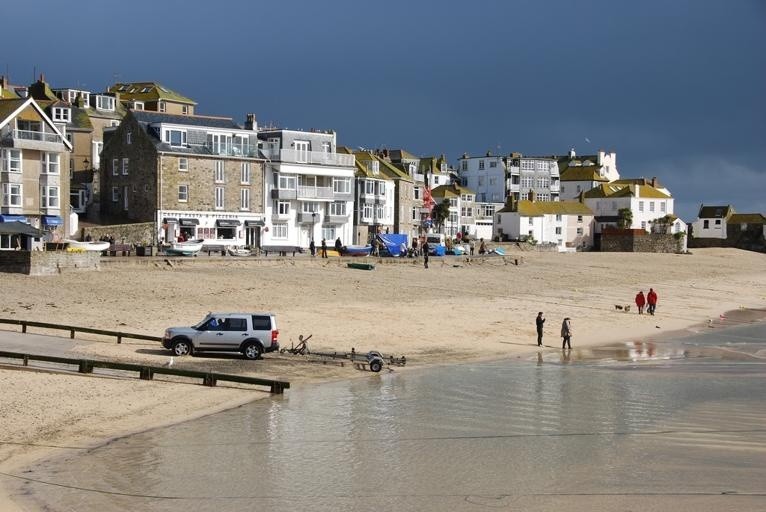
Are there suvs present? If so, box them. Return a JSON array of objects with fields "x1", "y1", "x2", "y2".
[{"x1": 160, "y1": 310, "x2": 281, "y2": 359}]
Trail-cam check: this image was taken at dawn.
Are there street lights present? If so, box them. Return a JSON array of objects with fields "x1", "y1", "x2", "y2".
[{"x1": 308, "y1": 211, "x2": 316, "y2": 256}]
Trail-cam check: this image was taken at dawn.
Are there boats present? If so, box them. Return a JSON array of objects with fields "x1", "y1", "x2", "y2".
[
  {"x1": 342, "y1": 241, "x2": 371, "y2": 256},
  {"x1": 449, "y1": 244, "x2": 505, "y2": 256},
  {"x1": 228, "y1": 248, "x2": 255, "y2": 257},
  {"x1": 320, "y1": 249, "x2": 339, "y2": 257},
  {"x1": 162, "y1": 239, "x2": 205, "y2": 258},
  {"x1": 347, "y1": 262, "x2": 376, "y2": 272},
  {"x1": 68, "y1": 239, "x2": 111, "y2": 252}
]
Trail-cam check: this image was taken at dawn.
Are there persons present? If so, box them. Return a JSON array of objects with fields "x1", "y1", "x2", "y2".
[
  {"x1": 322, "y1": 239, "x2": 328, "y2": 258},
  {"x1": 370, "y1": 238, "x2": 381, "y2": 256},
  {"x1": 536, "y1": 312, "x2": 545, "y2": 346},
  {"x1": 423, "y1": 242, "x2": 429, "y2": 255},
  {"x1": 470, "y1": 239, "x2": 475, "y2": 255},
  {"x1": 647, "y1": 288, "x2": 657, "y2": 316},
  {"x1": 561, "y1": 318, "x2": 570, "y2": 349},
  {"x1": 635, "y1": 291, "x2": 645, "y2": 314},
  {"x1": 336, "y1": 237, "x2": 342, "y2": 251},
  {"x1": 310, "y1": 241, "x2": 315, "y2": 256},
  {"x1": 400, "y1": 242, "x2": 407, "y2": 257},
  {"x1": 480, "y1": 238, "x2": 485, "y2": 254},
  {"x1": 218, "y1": 319, "x2": 224, "y2": 326}
]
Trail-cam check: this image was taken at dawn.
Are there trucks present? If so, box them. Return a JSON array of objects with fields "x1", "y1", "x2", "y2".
[{"x1": 422, "y1": 233, "x2": 446, "y2": 256}]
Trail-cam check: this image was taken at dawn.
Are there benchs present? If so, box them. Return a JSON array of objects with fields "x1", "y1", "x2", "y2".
[
  {"x1": 102, "y1": 244, "x2": 130, "y2": 256},
  {"x1": 201, "y1": 243, "x2": 225, "y2": 257},
  {"x1": 261, "y1": 245, "x2": 305, "y2": 257}
]
